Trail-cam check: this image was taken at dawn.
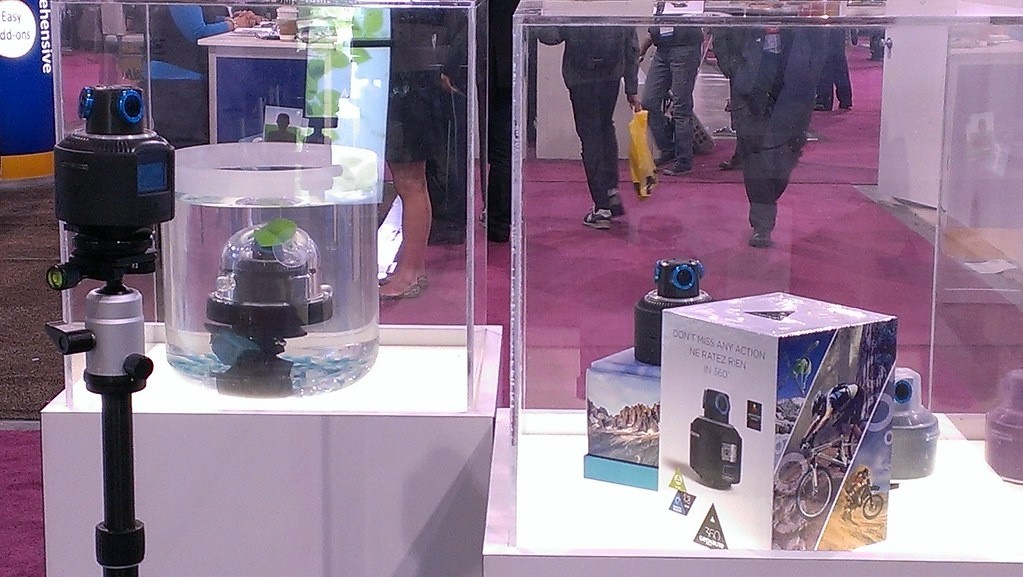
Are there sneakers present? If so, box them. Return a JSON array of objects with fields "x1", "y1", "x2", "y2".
[
  {"x1": 606, "y1": 188, "x2": 622, "y2": 215},
  {"x1": 583, "y1": 202, "x2": 612, "y2": 228},
  {"x1": 664, "y1": 161, "x2": 693, "y2": 176},
  {"x1": 654, "y1": 154, "x2": 675, "y2": 168}
]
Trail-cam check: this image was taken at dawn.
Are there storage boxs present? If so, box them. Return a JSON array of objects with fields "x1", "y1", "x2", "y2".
[{"x1": 584, "y1": 292, "x2": 898, "y2": 552}]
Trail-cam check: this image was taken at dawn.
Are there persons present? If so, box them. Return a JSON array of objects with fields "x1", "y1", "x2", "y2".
[
  {"x1": 710, "y1": 1, "x2": 828, "y2": 247},
  {"x1": 636, "y1": 0, "x2": 704, "y2": 175},
  {"x1": 534, "y1": 21, "x2": 641, "y2": 228},
  {"x1": 142, "y1": 2, "x2": 263, "y2": 149},
  {"x1": 800, "y1": 382, "x2": 864, "y2": 465},
  {"x1": 375, "y1": 0, "x2": 520, "y2": 300},
  {"x1": 865, "y1": 29, "x2": 884, "y2": 62},
  {"x1": 815, "y1": 26, "x2": 853, "y2": 112}
]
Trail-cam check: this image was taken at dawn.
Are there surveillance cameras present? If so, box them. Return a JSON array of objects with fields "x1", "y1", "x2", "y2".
[{"x1": 205, "y1": 222, "x2": 333, "y2": 329}]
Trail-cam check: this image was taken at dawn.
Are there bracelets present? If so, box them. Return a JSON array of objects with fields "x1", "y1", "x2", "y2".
[{"x1": 229, "y1": 18, "x2": 236, "y2": 31}]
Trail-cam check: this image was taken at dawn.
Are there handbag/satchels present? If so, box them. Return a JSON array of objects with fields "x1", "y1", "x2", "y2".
[{"x1": 626, "y1": 105, "x2": 659, "y2": 198}]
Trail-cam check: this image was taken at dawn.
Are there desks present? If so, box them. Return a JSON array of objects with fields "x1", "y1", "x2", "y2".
[{"x1": 198, "y1": 22, "x2": 307, "y2": 144}]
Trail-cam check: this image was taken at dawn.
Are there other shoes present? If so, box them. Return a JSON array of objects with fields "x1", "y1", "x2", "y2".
[
  {"x1": 814, "y1": 103, "x2": 827, "y2": 111},
  {"x1": 379, "y1": 275, "x2": 429, "y2": 289},
  {"x1": 487, "y1": 221, "x2": 510, "y2": 243},
  {"x1": 719, "y1": 154, "x2": 742, "y2": 170},
  {"x1": 379, "y1": 282, "x2": 420, "y2": 302},
  {"x1": 867, "y1": 55, "x2": 881, "y2": 61},
  {"x1": 839, "y1": 101, "x2": 850, "y2": 109},
  {"x1": 748, "y1": 228, "x2": 771, "y2": 247},
  {"x1": 427, "y1": 219, "x2": 465, "y2": 244}
]
